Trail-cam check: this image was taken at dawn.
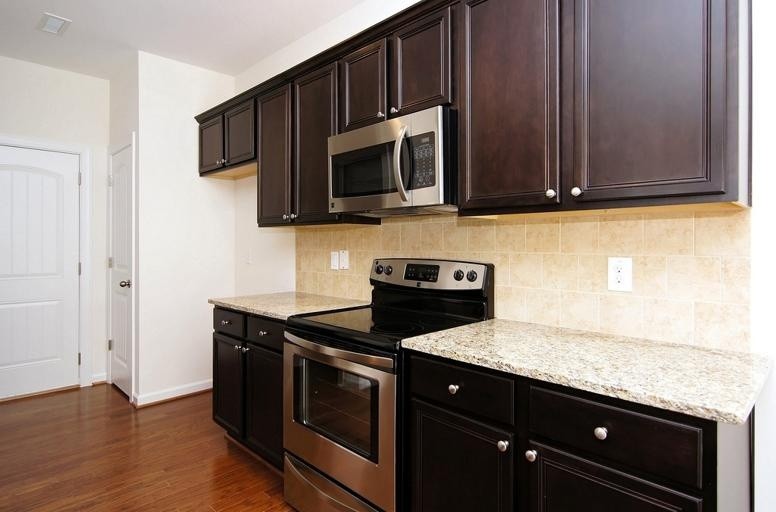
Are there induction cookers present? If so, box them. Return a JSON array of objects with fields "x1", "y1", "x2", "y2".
[{"x1": 288, "y1": 304, "x2": 480, "y2": 339}]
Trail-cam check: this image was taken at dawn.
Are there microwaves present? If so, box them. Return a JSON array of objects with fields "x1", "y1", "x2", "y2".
[{"x1": 326, "y1": 105, "x2": 458, "y2": 214}]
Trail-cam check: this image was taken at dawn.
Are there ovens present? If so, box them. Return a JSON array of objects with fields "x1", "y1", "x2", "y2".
[{"x1": 281, "y1": 327, "x2": 397, "y2": 511}]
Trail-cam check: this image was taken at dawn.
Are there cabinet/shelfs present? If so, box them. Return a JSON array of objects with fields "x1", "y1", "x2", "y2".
[
  {"x1": 463, "y1": 0, "x2": 752, "y2": 220},
  {"x1": 257, "y1": 61, "x2": 337, "y2": 228},
  {"x1": 194, "y1": 99, "x2": 258, "y2": 179},
  {"x1": 213, "y1": 306, "x2": 283, "y2": 471},
  {"x1": 337, "y1": 7, "x2": 452, "y2": 128},
  {"x1": 397, "y1": 347, "x2": 714, "y2": 512}
]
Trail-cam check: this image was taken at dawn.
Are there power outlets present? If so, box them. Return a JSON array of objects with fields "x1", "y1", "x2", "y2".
[{"x1": 607, "y1": 257, "x2": 633, "y2": 292}]
